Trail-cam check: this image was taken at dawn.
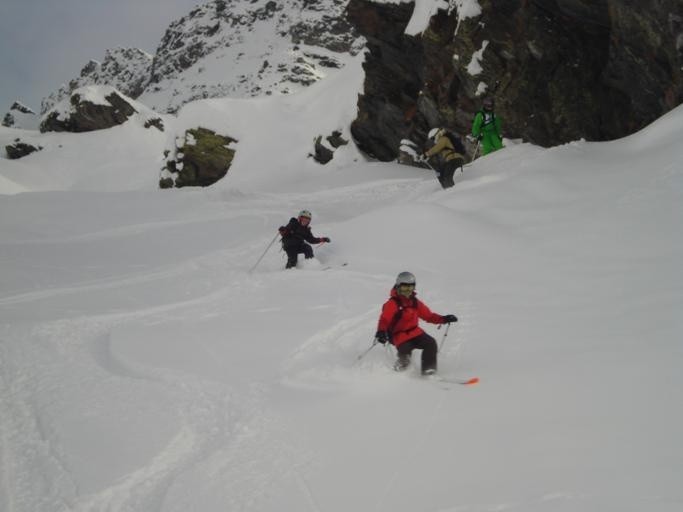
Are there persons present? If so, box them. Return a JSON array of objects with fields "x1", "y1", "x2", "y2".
[
  {"x1": 372, "y1": 270, "x2": 458, "y2": 377},
  {"x1": 470, "y1": 95, "x2": 503, "y2": 157},
  {"x1": 396, "y1": 108, "x2": 419, "y2": 164},
  {"x1": 422, "y1": 126, "x2": 465, "y2": 188},
  {"x1": 277, "y1": 209, "x2": 331, "y2": 269}
]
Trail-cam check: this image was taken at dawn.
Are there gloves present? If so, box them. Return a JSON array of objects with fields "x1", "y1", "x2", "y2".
[
  {"x1": 376, "y1": 330, "x2": 388, "y2": 344},
  {"x1": 420, "y1": 151, "x2": 431, "y2": 162},
  {"x1": 498, "y1": 134, "x2": 503, "y2": 142},
  {"x1": 320, "y1": 237, "x2": 330, "y2": 243},
  {"x1": 476, "y1": 135, "x2": 482, "y2": 142},
  {"x1": 279, "y1": 226, "x2": 288, "y2": 234},
  {"x1": 443, "y1": 314, "x2": 458, "y2": 323}
]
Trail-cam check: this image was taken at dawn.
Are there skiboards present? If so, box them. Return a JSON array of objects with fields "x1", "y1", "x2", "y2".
[{"x1": 386, "y1": 357, "x2": 479, "y2": 390}]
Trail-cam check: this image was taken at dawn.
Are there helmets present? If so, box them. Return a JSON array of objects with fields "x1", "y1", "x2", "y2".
[
  {"x1": 427, "y1": 127, "x2": 441, "y2": 144},
  {"x1": 483, "y1": 98, "x2": 495, "y2": 112},
  {"x1": 297, "y1": 209, "x2": 312, "y2": 226},
  {"x1": 395, "y1": 271, "x2": 417, "y2": 286}
]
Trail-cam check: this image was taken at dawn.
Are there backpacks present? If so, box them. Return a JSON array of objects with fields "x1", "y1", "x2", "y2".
[{"x1": 442, "y1": 132, "x2": 466, "y2": 155}]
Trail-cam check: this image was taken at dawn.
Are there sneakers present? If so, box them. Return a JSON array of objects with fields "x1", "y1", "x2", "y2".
[{"x1": 422, "y1": 369, "x2": 437, "y2": 377}]
